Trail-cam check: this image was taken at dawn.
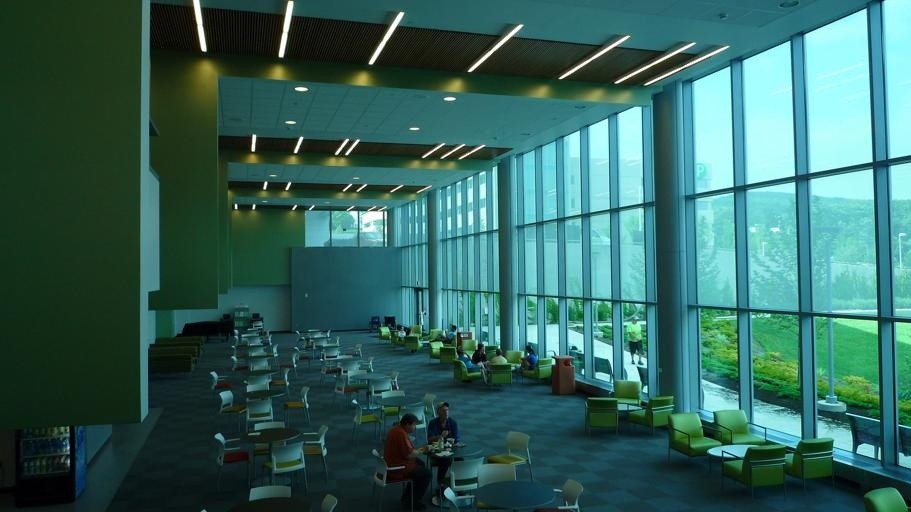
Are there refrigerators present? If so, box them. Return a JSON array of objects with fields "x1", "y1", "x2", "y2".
[{"x1": 15, "y1": 426, "x2": 88, "y2": 508}]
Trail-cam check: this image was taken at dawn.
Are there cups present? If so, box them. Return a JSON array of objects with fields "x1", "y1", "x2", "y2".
[{"x1": 425, "y1": 434, "x2": 457, "y2": 457}]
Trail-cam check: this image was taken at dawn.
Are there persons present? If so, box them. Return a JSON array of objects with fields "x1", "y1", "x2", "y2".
[
  {"x1": 428, "y1": 401, "x2": 462, "y2": 484},
  {"x1": 628, "y1": 315, "x2": 646, "y2": 365},
  {"x1": 457, "y1": 343, "x2": 508, "y2": 386},
  {"x1": 380, "y1": 414, "x2": 433, "y2": 511},
  {"x1": 523, "y1": 345, "x2": 539, "y2": 370},
  {"x1": 395, "y1": 324, "x2": 457, "y2": 353}
]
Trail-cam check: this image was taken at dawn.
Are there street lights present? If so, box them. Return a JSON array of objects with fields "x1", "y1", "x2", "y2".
[
  {"x1": 898, "y1": 233, "x2": 906, "y2": 270},
  {"x1": 815, "y1": 226, "x2": 845, "y2": 413}
]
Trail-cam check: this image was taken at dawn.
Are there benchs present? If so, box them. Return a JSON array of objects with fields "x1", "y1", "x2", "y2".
[{"x1": 148, "y1": 336, "x2": 205, "y2": 376}]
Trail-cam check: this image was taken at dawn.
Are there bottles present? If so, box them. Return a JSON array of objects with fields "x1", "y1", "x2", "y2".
[{"x1": 21, "y1": 426, "x2": 71, "y2": 475}]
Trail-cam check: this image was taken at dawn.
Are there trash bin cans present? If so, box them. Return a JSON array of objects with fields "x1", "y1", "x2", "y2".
[{"x1": 551, "y1": 356, "x2": 576, "y2": 395}]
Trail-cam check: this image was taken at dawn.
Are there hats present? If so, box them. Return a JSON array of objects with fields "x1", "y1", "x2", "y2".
[{"x1": 437, "y1": 402, "x2": 449, "y2": 410}]
